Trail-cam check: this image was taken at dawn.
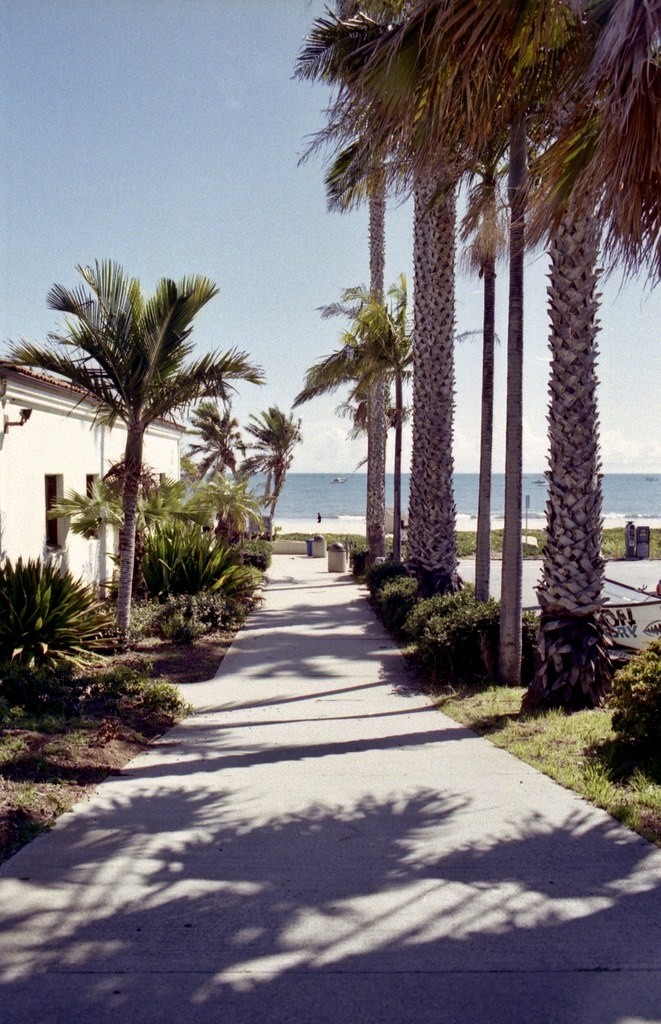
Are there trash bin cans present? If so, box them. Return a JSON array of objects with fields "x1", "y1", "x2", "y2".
[
  {"x1": 305, "y1": 539, "x2": 314, "y2": 556},
  {"x1": 311, "y1": 535, "x2": 325, "y2": 558},
  {"x1": 328, "y1": 542, "x2": 346, "y2": 573}
]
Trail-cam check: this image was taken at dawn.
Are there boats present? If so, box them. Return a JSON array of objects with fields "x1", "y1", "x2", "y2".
[
  {"x1": 644, "y1": 477, "x2": 659, "y2": 482},
  {"x1": 330, "y1": 478, "x2": 346, "y2": 483},
  {"x1": 531, "y1": 479, "x2": 545, "y2": 484}
]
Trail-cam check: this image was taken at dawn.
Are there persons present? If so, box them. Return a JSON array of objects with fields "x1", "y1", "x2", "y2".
[{"x1": 316, "y1": 512, "x2": 321, "y2": 523}]
